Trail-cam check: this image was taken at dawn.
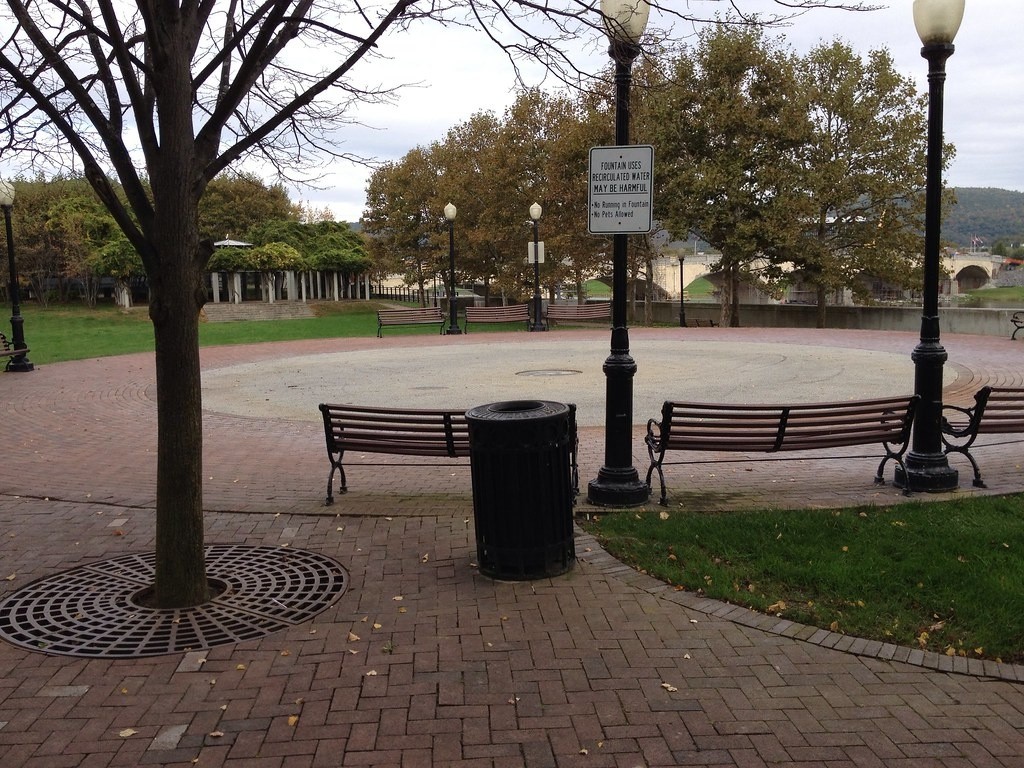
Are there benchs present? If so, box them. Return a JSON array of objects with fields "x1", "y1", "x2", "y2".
[
  {"x1": 545, "y1": 302, "x2": 614, "y2": 330},
  {"x1": 462, "y1": 304, "x2": 532, "y2": 334},
  {"x1": 319, "y1": 402, "x2": 579, "y2": 505},
  {"x1": 0, "y1": 333, "x2": 32, "y2": 372},
  {"x1": 684, "y1": 318, "x2": 720, "y2": 328},
  {"x1": 374, "y1": 307, "x2": 445, "y2": 339},
  {"x1": 635, "y1": 395, "x2": 923, "y2": 499},
  {"x1": 941, "y1": 387, "x2": 1024, "y2": 490},
  {"x1": 1009, "y1": 310, "x2": 1024, "y2": 340}
]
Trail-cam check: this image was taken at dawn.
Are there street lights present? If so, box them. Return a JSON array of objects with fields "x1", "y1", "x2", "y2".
[
  {"x1": 894, "y1": 0, "x2": 966, "y2": 490},
  {"x1": 0, "y1": 179, "x2": 34, "y2": 373},
  {"x1": 587, "y1": 0, "x2": 651, "y2": 508},
  {"x1": 677, "y1": 247, "x2": 687, "y2": 327},
  {"x1": 530, "y1": 202, "x2": 546, "y2": 332},
  {"x1": 444, "y1": 202, "x2": 462, "y2": 335}
]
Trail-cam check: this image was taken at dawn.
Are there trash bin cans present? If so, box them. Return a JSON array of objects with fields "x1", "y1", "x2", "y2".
[{"x1": 459, "y1": 400, "x2": 578, "y2": 582}]
